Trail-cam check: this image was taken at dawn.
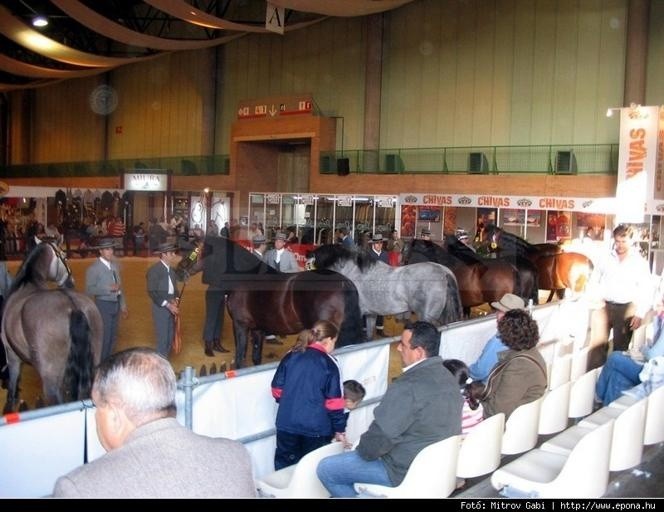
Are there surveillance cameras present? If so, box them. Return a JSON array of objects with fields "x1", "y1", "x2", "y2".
[{"x1": 606, "y1": 108, "x2": 614, "y2": 118}]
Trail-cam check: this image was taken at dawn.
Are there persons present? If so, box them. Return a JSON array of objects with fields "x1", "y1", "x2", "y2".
[
  {"x1": 591, "y1": 225, "x2": 656, "y2": 351},
  {"x1": 316, "y1": 320, "x2": 463, "y2": 498},
  {"x1": 443, "y1": 359, "x2": 482, "y2": 433},
  {"x1": 341, "y1": 379, "x2": 365, "y2": 429},
  {"x1": 0, "y1": 213, "x2": 486, "y2": 361},
  {"x1": 592, "y1": 277, "x2": 663, "y2": 410},
  {"x1": 478, "y1": 308, "x2": 549, "y2": 430},
  {"x1": 51, "y1": 346, "x2": 259, "y2": 499},
  {"x1": 270, "y1": 318, "x2": 355, "y2": 470},
  {"x1": 467, "y1": 293, "x2": 526, "y2": 383}
]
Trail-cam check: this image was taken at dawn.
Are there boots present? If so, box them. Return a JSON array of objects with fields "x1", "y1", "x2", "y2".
[
  {"x1": 215, "y1": 339, "x2": 229, "y2": 353},
  {"x1": 204, "y1": 341, "x2": 214, "y2": 356}
]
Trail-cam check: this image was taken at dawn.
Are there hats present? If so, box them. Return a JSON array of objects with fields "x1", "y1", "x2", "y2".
[
  {"x1": 253, "y1": 234, "x2": 387, "y2": 245},
  {"x1": 153, "y1": 243, "x2": 177, "y2": 254},
  {"x1": 91, "y1": 238, "x2": 119, "y2": 250},
  {"x1": 491, "y1": 293, "x2": 525, "y2": 312}
]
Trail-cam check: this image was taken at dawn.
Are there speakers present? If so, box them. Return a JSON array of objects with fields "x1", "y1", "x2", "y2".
[{"x1": 337, "y1": 158, "x2": 349, "y2": 176}]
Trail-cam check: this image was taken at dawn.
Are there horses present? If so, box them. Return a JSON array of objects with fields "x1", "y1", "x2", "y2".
[
  {"x1": 303, "y1": 242, "x2": 464, "y2": 341},
  {"x1": 173, "y1": 235, "x2": 362, "y2": 369},
  {"x1": 0, "y1": 233, "x2": 105, "y2": 413},
  {"x1": 400, "y1": 220, "x2": 596, "y2": 318}
]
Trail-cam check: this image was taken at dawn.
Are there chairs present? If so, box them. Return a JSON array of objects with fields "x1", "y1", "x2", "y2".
[{"x1": 254, "y1": 333, "x2": 663, "y2": 499}]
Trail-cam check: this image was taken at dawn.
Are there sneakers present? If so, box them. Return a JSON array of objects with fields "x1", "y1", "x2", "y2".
[
  {"x1": 267, "y1": 338, "x2": 282, "y2": 345},
  {"x1": 376, "y1": 330, "x2": 389, "y2": 337}
]
